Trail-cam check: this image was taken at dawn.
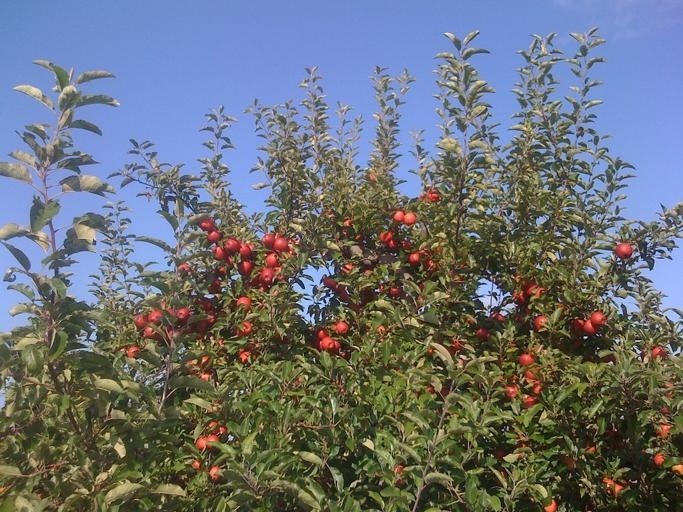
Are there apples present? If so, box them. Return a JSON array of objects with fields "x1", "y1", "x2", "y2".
[{"x1": 125, "y1": 185, "x2": 683, "y2": 512}]
